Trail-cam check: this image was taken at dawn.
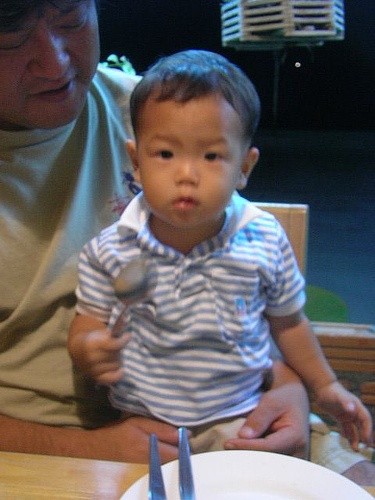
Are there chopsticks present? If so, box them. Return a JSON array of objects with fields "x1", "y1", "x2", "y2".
[{"x1": 148, "y1": 427, "x2": 195, "y2": 500}]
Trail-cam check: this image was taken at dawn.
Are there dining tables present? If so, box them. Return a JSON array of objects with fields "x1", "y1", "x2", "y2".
[{"x1": 0, "y1": 449, "x2": 375, "y2": 500}]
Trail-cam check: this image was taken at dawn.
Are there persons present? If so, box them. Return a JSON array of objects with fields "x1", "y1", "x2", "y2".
[
  {"x1": 1, "y1": 0, "x2": 374, "y2": 486},
  {"x1": 66, "y1": 49, "x2": 375, "y2": 454}
]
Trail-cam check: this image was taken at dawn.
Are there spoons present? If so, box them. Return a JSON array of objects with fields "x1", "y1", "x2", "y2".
[{"x1": 94, "y1": 260, "x2": 148, "y2": 390}]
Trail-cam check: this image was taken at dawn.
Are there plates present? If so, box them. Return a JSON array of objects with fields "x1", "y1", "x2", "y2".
[{"x1": 120, "y1": 450, "x2": 375, "y2": 500}]
